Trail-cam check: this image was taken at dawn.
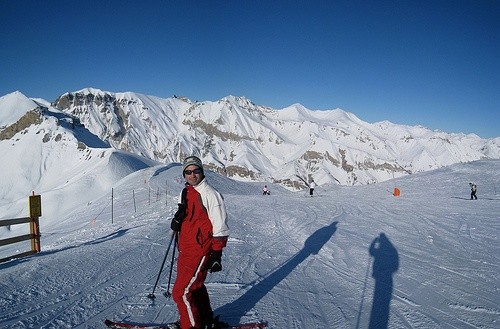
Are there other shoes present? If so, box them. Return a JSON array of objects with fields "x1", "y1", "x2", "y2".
[{"x1": 167, "y1": 320, "x2": 181, "y2": 329}]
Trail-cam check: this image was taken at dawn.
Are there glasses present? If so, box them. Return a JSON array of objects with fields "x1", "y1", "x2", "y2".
[{"x1": 183, "y1": 169, "x2": 201, "y2": 175}]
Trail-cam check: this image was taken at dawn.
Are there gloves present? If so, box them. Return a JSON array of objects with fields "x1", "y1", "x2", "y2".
[
  {"x1": 170, "y1": 207, "x2": 185, "y2": 231},
  {"x1": 207, "y1": 250, "x2": 222, "y2": 273}
]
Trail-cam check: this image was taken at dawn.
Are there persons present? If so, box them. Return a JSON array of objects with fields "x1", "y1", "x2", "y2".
[
  {"x1": 263, "y1": 185, "x2": 268, "y2": 195},
  {"x1": 170, "y1": 155, "x2": 230, "y2": 329},
  {"x1": 468, "y1": 182, "x2": 478, "y2": 200},
  {"x1": 309, "y1": 180, "x2": 315, "y2": 197}
]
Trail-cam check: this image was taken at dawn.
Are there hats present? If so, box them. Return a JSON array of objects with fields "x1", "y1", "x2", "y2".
[{"x1": 182, "y1": 156, "x2": 203, "y2": 178}]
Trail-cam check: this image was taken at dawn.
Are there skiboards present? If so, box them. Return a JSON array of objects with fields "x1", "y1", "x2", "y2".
[
  {"x1": 103, "y1": 314, "x2": 221, "y2": 329},
  {"x1": 204, "y1": 322, "x2": 269, "y2": 329}
]
